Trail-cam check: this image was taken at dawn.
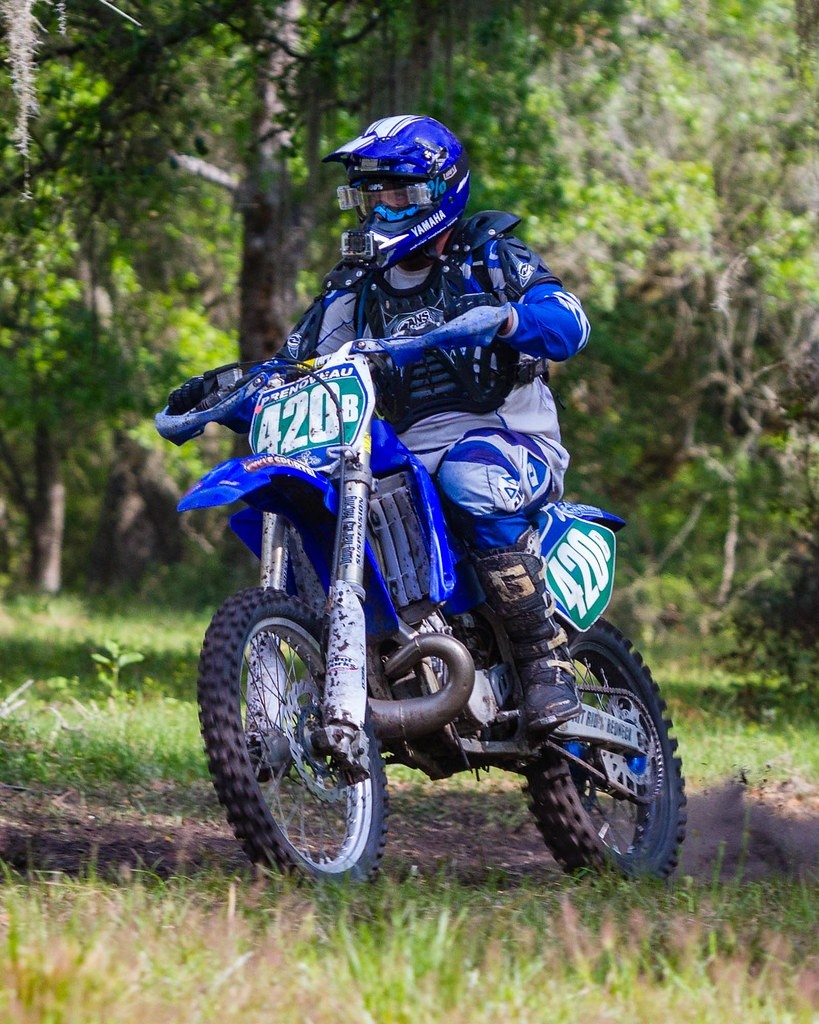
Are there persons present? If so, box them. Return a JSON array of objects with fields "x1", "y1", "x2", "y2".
[{"x1": 167, "y1": 114, "x2": 592, "y2": 729}]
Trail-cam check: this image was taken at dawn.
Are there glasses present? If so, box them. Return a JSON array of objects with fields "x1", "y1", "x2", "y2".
[{"x1": 356, "y1": 182, "x2": 431, "y2": 216}]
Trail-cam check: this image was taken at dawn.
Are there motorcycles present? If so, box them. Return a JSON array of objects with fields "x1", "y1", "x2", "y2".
[{"x1": 152, "y1": 301, "x2": 685, "y2": 892}]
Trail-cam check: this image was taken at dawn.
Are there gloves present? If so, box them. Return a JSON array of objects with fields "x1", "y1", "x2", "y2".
[{"x1": 168, "y1": 374, "x2": 203, "y2": 416}]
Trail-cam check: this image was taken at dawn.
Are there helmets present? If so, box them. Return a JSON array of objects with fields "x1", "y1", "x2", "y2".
[{"x1": 322, "y1": 115, "x2": 470, "y2": 267}]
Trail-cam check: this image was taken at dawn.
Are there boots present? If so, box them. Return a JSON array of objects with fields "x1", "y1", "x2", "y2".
[{"x1": 467, "y1": 538, "x2": 582, "y2": 732}]
